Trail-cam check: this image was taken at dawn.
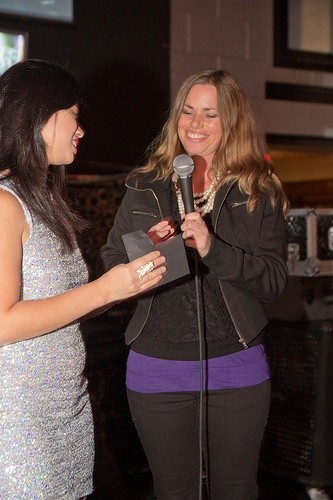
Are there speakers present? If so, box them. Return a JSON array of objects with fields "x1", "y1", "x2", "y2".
[{"x1": 263, "y1": 319, "x2": 333, "y2": 489}]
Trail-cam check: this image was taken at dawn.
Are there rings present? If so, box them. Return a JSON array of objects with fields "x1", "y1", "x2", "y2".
[{"x1": 135, "y1": 261, "x2": 155, "y2": 280}]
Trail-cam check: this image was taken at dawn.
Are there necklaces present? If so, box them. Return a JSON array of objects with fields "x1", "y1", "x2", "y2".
[{"x1": 174, "y1": 168, "x2": 221, "y2": 217}]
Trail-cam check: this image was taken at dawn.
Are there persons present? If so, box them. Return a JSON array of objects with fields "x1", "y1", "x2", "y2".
[
  {"x1": 0, "y1": 60, "x2": 167, "y2": 500},
  {"x1": 99, "y1": 68, "x2": 289, "y2": 500}
]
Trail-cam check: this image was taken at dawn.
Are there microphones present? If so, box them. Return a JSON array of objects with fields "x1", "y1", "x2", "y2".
[{"x1": 173, "y1": 154, "x2": 195, "y2": 215}]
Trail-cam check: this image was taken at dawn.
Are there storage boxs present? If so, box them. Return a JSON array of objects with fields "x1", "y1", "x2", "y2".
[{"x1": 285, "y1": 208, "x2": 333, "y2": 278}]
